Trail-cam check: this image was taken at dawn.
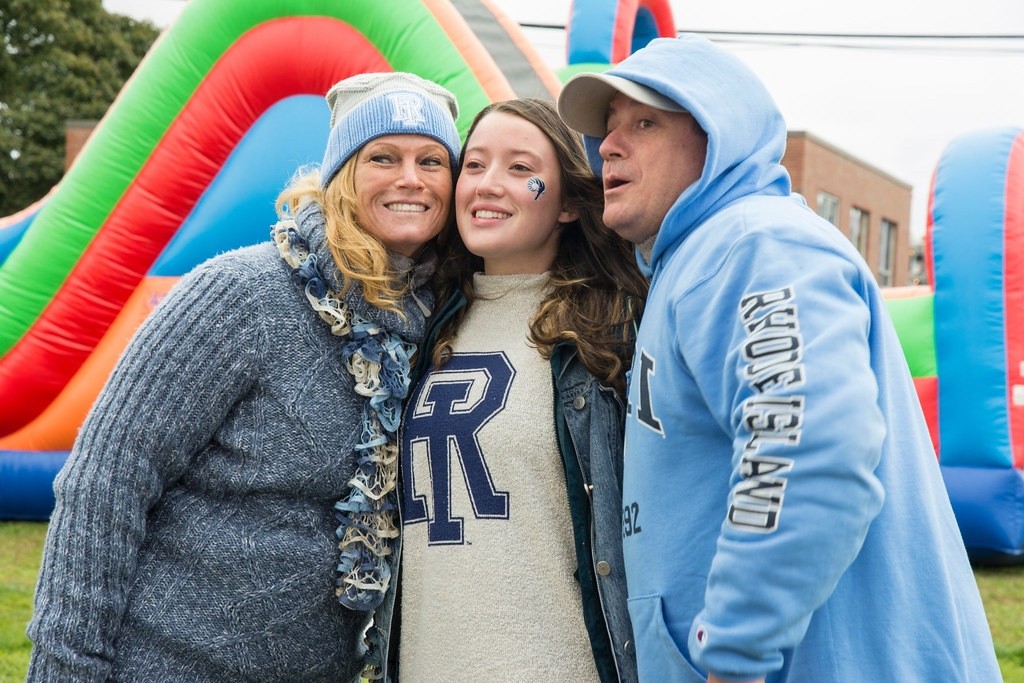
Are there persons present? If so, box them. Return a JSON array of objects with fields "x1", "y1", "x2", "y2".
[
  {"x1": 26, "y1": 72, "x2": 461, "y2": 683},
  {"x1": 558, "y1": 35, "x2": 1001, "y2": 683},
  {"x1": 368, "y1": 98, "x2": 638, "y2": 683}
]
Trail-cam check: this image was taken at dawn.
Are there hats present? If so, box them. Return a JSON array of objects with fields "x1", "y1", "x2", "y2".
[
  {"x1": 317, "y1": 71, "x2": 462, "y2": 192},
  {"x1": 556, "y1": 71, "x2": 690, "y2": 137}
]
